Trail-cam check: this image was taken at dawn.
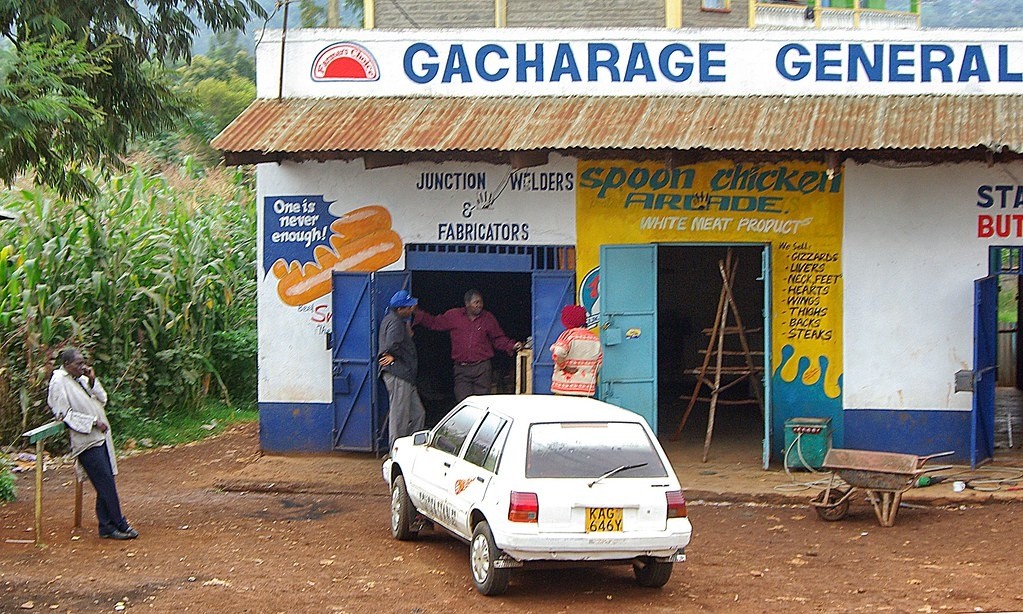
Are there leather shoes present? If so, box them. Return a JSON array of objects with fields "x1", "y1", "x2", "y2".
[
  {"x1": 99, "y1": 529, "x2": 130, "y2": 540},
  {"x1": 123, "y1": 527, "x2": 139, "y2": 538}
]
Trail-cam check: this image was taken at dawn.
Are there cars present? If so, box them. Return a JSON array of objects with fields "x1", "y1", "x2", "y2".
[{"x1": 381, "y1": 393, "x2": 692, "y2": 597}]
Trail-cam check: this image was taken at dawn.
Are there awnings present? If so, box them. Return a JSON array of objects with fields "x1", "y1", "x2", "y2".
[{"x1": 211, "y1": 95, "x2": 1023, "y2": 155}]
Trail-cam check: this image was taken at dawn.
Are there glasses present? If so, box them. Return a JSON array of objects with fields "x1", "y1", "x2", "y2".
[{"x1": 400, "y1": 306, "x2": 412, "y2": 310}]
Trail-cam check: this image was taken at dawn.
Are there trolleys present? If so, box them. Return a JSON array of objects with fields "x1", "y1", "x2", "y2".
[{"x1": 808, "y1": 447, "x2": 955, "y2": 528}]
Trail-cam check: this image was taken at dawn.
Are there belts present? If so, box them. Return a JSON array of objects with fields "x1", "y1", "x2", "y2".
[{"x1": 453, "y1": 360, "x2": 485, "y2": 367}]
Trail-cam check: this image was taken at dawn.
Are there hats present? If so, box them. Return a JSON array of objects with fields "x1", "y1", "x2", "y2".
[
  {"x1": 561, "y1": 305, "x2": 587, "y2": 328},
  {"x1": 390, "y1": 290, "x2": 419, "y2": 309}
]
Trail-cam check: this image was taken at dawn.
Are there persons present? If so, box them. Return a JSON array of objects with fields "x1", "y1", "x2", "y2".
[
  {"x1": 378, "y1": 290, "x2": 426, "y2": 458},
  {"x1": 411, "y1": 289, "x2": 522, "y2": 402},
  {"x1": 47, "y1": 349, "x2": 139, "y2": 539},
  {"x1": 550, "y1": 306, "x2": 602, "y2": 397}
]
